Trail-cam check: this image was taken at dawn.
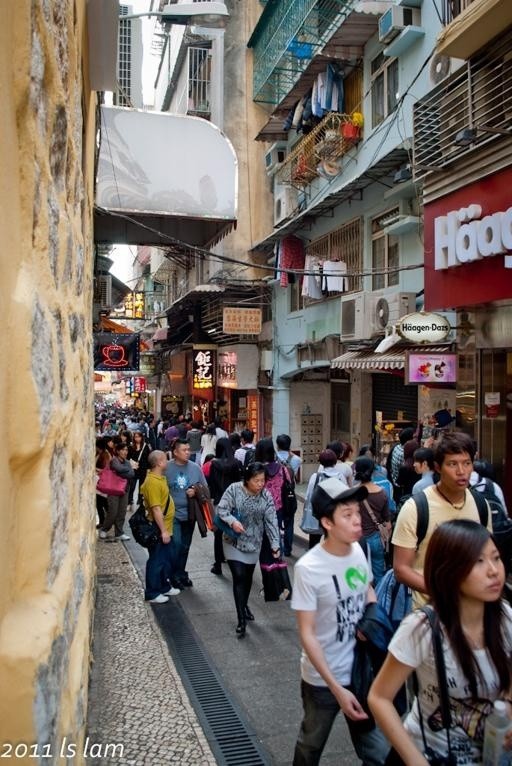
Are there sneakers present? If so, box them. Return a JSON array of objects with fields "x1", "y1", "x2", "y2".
[
  {"x1": 163, "y1": 587, "x2": 181, "y2": 595},
  {"x1": 96, "y1": 523, "x2": 130, "y2": 541},
  {"x1": 145, "y1": 594, "x2": 169, "y2": 603}
]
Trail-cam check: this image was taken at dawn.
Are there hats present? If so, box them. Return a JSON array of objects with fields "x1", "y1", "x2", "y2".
[
  {"x1": 434, "y1": 410, "x2": 455, "y2": 427},
  {"x1": 310, "y1": 478, "x2": 369, "y2": 516}
]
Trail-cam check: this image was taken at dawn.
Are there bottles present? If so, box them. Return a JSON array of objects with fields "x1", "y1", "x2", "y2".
[{"x1": 482, "y1": 697, "x2": 511, "y2": 765}]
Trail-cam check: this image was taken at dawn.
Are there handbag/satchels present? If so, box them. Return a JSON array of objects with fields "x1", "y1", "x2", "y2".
[
  {"x1": 376, "y1": 525, "x2": 390, "y2": 553},
  {"x1": 96, "y1": 459, "x2": 128, "y2": 495},
  {"x1": 212, "y1": 483, "x2": 241, "y2": 539},
  {"x1": 385, "y1": 746, "x2": 457, "y2": 766},
  {"x1": 95, "y1": 470, "x2": 108, "y2": 498},
  {"x1": 195, "y1": 448, "x2": 203, "y2": 467},
  {"x1": 129, "y1": 459, "x2": 140, "y2": 470},
  {"x1": 129, "y1": 504, "x2": 160, "y2": 547},
  {"x1": 262, "y1": 549, "x2": 292, "y2": 601}
]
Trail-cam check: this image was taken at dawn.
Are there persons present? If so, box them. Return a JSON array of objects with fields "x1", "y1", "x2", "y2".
[{"x1": 93, "y1": 399, "x2": 512, "y2": 766}]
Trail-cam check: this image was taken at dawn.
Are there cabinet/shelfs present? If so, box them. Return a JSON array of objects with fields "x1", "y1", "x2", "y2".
[{"x1": 300, "y1": 414, "x2": 323, "y2": 464}]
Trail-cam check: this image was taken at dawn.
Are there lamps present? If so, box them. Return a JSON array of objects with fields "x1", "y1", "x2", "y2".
[
  {"x1": 391, "y1": 163, "x2": 442, "y2": 185},
  {"x1": 453, "y1": 125, "x2": 510, "y2": 146},
  {"x1": 117, "y1": 3, "x2": 231, "y2": 29}
]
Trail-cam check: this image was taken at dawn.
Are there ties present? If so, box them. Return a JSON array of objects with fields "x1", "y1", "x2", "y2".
[{"x1": 179, "y1": 576, "x2": 192, "y2": 586}]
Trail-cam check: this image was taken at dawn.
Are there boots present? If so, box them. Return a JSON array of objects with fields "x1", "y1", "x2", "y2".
[
  {"x1": 245, "y1": 606, "x2": 254, "y2": 620},
  {"x1": 236, "y1": 606, "x2": 245, "y2": 633}
]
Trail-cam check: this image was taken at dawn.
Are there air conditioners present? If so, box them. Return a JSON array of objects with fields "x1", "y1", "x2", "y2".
[
  {"x1": 96, "y1": 275, "x2": 112, "y2": 310},
  {"x1": 265, "y1": 148, "x2": 286, "y2": 172},
  {"x1": 274, "y1": 188, "x2": 298, "y2": 227},
  {"x1": 428, "y1": 49, "x2": 465, "y2": 89},
  {"x1": 374, "y1": 292, "x2": 417, "y2": 335},
  {"x1": 340, "y1": 290, "x2": 383, "y2": 342},
  {"x1": 378, "y1": 4, "x2": 421, "y2": 46},
  {"x1": 104, "y1": 3, "x2": 144, "y2": 108}
]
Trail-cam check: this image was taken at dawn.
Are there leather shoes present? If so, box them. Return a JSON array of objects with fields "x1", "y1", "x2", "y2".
[
  {"x1": 211, "y1": 563, "x2": 222, "y2": 575},
  {"x1": 170, "y1": 576, "x2": 184, "y2": 589}
]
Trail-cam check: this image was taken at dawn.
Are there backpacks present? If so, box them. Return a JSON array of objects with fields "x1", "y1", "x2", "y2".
[
  {"x1": 276, "y1": 450, "x2": 295, "y2": 483},
  {"x1": 469, "y1": 476, "x2": 512, "y2": 579},
  {"x1": 242, "y1": 446, "x2": 256, "y2": 470},
  {"x1": 280, "y1": 468, "x2": 297, "y2": 530}
]
